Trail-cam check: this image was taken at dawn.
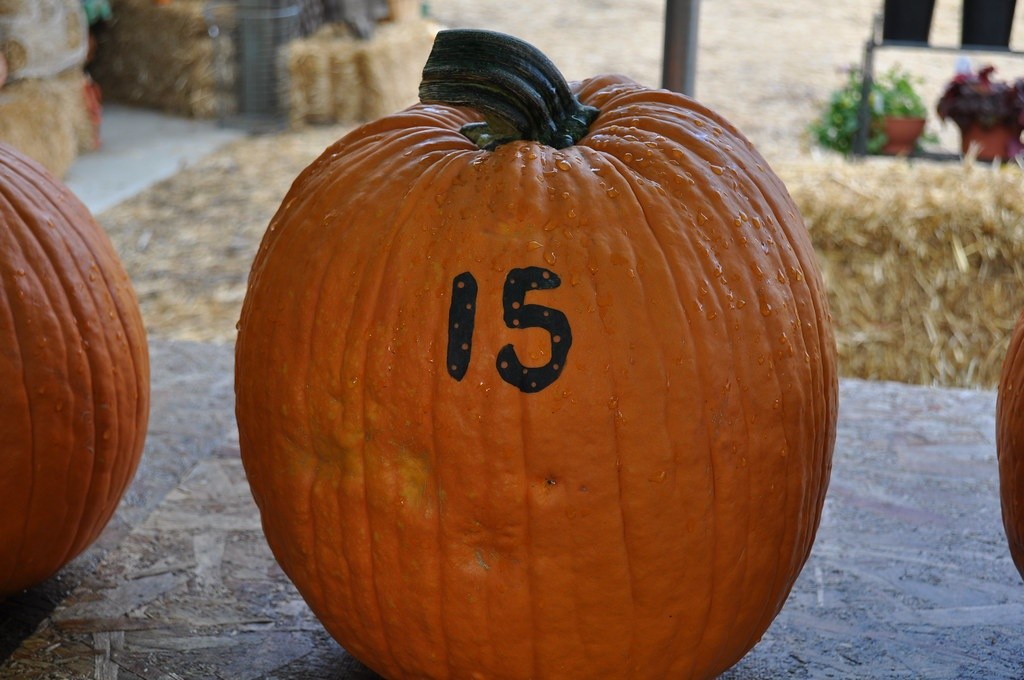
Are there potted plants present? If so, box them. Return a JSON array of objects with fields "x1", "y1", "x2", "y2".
[{"x1": 879, "y1": 68, "x2": 927, "y2": 154}]
